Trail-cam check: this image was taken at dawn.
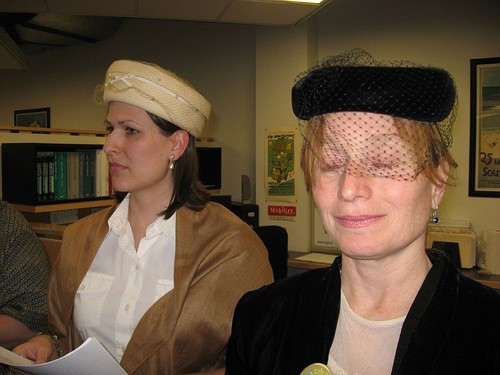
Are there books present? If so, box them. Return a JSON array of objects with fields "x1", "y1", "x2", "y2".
[
  {"x1": 29, "y1": 152, "x2": 79, "y2": 202},
  {"x1": 74, "y1": 149, "x2": 116, "y2": 198}
]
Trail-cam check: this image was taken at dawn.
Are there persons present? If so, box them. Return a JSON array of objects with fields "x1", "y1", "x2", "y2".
[
  {"x1": 10, "y1": 59, "x2": 274, "y2": 375},
  {"x1": 0, "y1": 199, "x2": 50, "y2": 350},
  {"x1": 225, "y1": 47, "x2": 500, "y2": 375}
]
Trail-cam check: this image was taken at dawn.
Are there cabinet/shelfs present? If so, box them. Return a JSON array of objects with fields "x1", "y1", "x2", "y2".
[{"x1": 0, "y1": 126, "x2": 222, "y2": 240}]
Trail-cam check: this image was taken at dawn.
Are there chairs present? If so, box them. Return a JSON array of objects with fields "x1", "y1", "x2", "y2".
[{"x1": 253, "y1": 225, "x2": 289, "y2": 283}]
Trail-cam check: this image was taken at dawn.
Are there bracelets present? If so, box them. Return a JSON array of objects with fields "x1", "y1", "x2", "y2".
[{"x1": 52, "y1": 335, "x2": 62, "y2": 358}]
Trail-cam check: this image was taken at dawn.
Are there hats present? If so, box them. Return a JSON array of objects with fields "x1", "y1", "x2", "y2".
[
  {"x1": 93, "y1": 59, "x2": 213, "y2": 139},
  {"x1": 290, "y1": 48, "x2": 457, "y2": 181}
]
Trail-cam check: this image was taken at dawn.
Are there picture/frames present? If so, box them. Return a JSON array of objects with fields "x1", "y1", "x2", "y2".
[
  {"x1": 469, "y1": 57, "x2": 500, "y2": 198},
  {"x1": 14, "y1": 107, "x2": 51, "y2": 133}
]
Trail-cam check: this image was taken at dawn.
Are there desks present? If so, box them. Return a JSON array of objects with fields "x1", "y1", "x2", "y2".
[{"x1": 286, "y1": 251, "x2": 500, "y2": 289}]
